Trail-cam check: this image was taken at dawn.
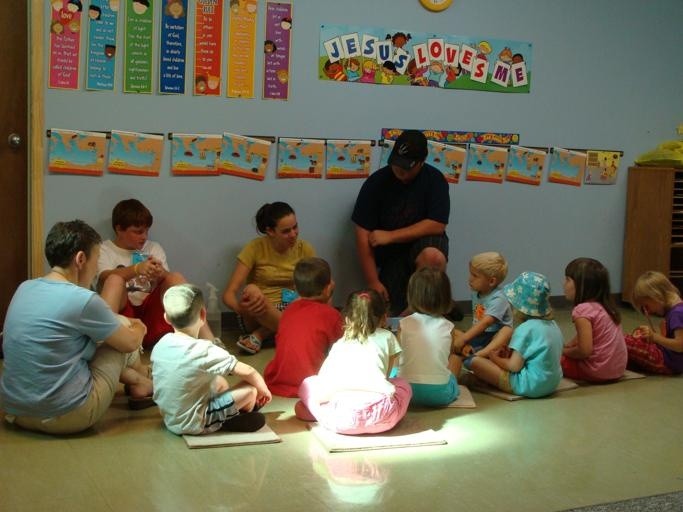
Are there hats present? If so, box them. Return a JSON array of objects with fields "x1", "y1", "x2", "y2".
[
  {"x1": 386, "y1": 129, "x2": 429, "y2": 170},
  {"x1": 505, "y1": 271, "x2": 552, "y2": 318}
]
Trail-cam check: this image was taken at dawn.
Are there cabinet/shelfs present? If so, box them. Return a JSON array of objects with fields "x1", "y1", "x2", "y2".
[{"x1": 621, "y1": 165, "x2": 682, "y2": 308}]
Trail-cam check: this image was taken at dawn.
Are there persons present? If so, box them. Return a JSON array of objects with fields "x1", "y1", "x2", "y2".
[
  {"x1": 90, "y1": 199, "x2": 227, "y2": 350},
  {"x1": 222, "y1": 201, "x2": 336, "y2": 354},
  {"x1": 263, "y1": 257, "x2": 345, "y2": 398},
  {"x1": 560, "y1": 257, "x2": 628, "y2": 382},
  {"x1": 453, "y1": 251, "x2": 513, "y2": 358},
  {"x1": 351, "y1": 130, "x2": 450, "y2": 315},
  {"x1": 150, "y1": 282, "x2": 272, "y2": 436},
  {"x1": 0, "y1": 219, "x2": 157, "y2": 435},
  {"x1": 396, "y1": 267, "x2": 462, "y2": 407},
  {"x1": 627, "y1": 271, "x2": 683, "y2": 377},
  {"x1": 294, "y1": 289, "x2": 413, "y2": 435},
  {"x1": 470, "y1": 271, "x2": 564, "y2": 399}
]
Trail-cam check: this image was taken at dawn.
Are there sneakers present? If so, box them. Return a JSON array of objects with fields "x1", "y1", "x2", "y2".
[{"x1": 219, "y1": 411, "x2": 267, "y2": 434}]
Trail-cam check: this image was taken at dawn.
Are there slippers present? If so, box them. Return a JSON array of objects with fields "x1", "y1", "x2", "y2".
[
  {"x1": 236, "y1": 333, "x2": 263, "y2": 354},
  {"x1": 126, "y1": 393, "x2": 157, "y2": 410}
]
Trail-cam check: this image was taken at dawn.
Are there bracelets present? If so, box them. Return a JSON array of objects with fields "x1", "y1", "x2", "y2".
[{"x1": 133, "y1": 262, "x2": 139, "y2": 276}]
